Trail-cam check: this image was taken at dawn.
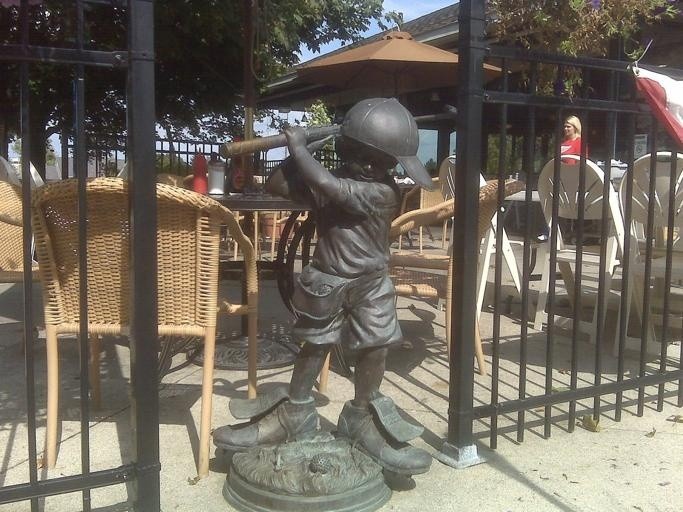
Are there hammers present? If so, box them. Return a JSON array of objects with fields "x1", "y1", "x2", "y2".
[{"x1": 219, "y1": 104, "x2": 458, "y2": 159}]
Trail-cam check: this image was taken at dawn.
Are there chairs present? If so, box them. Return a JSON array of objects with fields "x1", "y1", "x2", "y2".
[
  {"x1": 0, "y1": 153, "x2": 311, "y2": 479},
  {"x1": 318, "y1": 153, "x2": 683, "y2": 392}
]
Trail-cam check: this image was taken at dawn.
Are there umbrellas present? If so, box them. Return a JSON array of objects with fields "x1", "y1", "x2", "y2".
[{"x1": 295, "y1": 29, "x2": 512, "y2": 102}]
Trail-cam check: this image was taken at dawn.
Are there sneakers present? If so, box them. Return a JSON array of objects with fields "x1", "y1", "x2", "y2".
[{"x1": 537, "y1": 234, "x2": 548, "y2": 241}]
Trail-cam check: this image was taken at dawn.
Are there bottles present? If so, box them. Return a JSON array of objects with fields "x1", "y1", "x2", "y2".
[{"x1": 192, "y1": 147, "x2": 226, "y2": 195}]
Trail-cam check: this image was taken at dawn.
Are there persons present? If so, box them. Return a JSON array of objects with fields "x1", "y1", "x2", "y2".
[
  {"x1": 209, "y1": 100, "x2": 437, "y2": 477},
  {"x1": 560, "y1": 114, "x2": 589, "y2": 164}
]
